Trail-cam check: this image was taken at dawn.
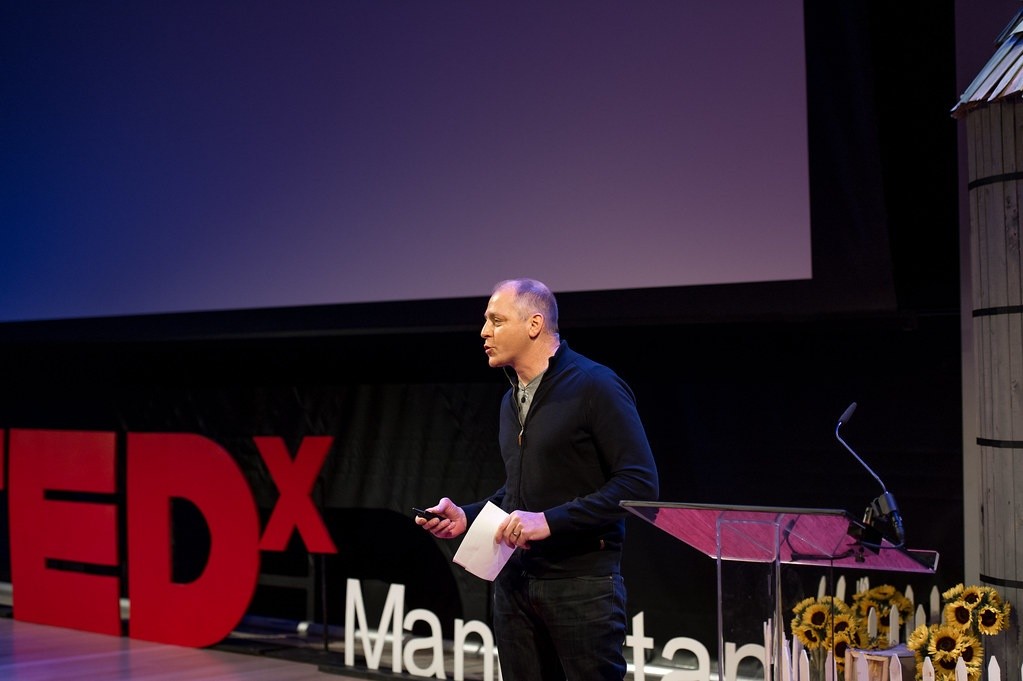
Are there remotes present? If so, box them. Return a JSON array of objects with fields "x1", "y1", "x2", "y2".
[{"x1": 412, "y1": 508, "x2": 453, "y2": 523}]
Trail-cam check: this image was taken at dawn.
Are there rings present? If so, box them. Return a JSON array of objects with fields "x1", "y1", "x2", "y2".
[{"x1": 512, "y1": 531, "x2": 519, "y2": 537}]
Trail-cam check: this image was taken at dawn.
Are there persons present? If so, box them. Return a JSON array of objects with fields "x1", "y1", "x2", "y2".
[{"x1": 415, "y1": 277, "x2": 660, "y2": 681}]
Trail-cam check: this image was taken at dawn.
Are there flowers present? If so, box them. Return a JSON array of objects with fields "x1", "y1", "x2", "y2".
[{"x1": 791, "y1": 582, "x2": 1010, "y2": 681}]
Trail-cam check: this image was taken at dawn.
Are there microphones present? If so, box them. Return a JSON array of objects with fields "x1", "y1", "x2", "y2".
[
  {"x1": 836, "y1": 402, "x2": 904, "y2": 539},
  {"x1": 521, "y1": 397, "x2": 525, "y2": 403}
]
